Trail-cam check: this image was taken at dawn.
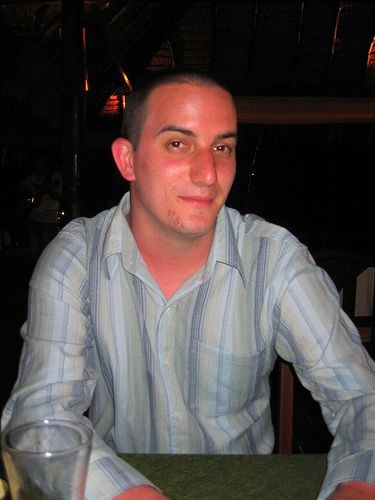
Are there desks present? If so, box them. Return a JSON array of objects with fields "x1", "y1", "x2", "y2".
[{"x1": 0, "y1": 454, "x2": 375, "y2": 500}]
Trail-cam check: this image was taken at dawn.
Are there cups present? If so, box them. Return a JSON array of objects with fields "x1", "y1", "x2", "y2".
[{"x1": 0, "y1": 419, "x2": 94, "y2": 500}]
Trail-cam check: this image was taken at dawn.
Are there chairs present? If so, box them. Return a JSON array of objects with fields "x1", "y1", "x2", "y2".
[
  {"x1": 271, "y1": 361, "x2": 293, "y2": 453},
  {"x1": 340, "y1": 266, "x2": 375, "y2": 360}
]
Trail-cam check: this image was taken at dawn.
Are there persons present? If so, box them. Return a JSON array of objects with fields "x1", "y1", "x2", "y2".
[{"x1": 0, "y1": 69, "x2": 375, "y2": 500}]
